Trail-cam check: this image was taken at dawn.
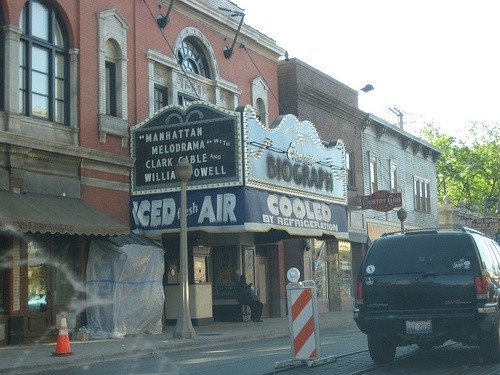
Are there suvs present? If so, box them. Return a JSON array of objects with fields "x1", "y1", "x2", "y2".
[{"x1": 353, "y1": 227, "x2": 500, "y2": 364}]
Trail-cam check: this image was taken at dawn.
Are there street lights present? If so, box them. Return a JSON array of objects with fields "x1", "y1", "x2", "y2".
[
  {"x1": 397, "y1": 207, "x2": 408, "y2": 234},
  {"x1": 172, "y1": 157, "x2": 197, "y2": 340}
]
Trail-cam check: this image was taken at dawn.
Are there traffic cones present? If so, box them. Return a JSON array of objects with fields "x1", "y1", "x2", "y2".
[{"x1": 51, "y1": 314, "x2": 74, "y2": 355}]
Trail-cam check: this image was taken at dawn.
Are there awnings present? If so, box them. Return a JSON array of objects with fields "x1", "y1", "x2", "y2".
[{"x1": 1, "y1": 189, "x2": 131, "y2": 238}]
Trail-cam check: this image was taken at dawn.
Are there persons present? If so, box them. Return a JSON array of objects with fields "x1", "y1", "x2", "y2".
[{"x1": 236, "y1": 275, "x2": 265, "y2": 323}]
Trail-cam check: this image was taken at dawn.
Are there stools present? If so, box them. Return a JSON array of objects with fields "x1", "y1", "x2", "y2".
[{"x1": 240, "y1": 302, "x2": 250, "y2": 322}]
